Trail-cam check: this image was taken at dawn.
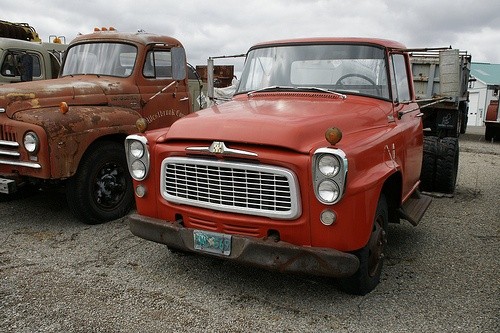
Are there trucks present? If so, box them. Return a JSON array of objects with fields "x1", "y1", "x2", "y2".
[{"x1": 1, "y1": 16, "x2": 473, "y2": 294}]
